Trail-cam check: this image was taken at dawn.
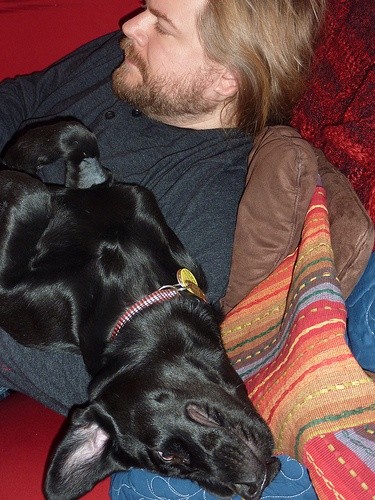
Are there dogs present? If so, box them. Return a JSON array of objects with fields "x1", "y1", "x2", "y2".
[{"x1": 0, "y1": 120, "x2": 282, "y2": 500}]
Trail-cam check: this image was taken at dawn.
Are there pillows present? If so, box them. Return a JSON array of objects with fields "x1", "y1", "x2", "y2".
[
  {"x1": 213, "y1": 126, "x2": 321, "y2": 317},
  {"x1": 305, "y1": 151, "x2": 374, "y2": 300}
]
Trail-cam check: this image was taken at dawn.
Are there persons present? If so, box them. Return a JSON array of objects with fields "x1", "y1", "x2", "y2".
[{"x1": 0, "y1": 0, "x2": 326, "y2": 417}]
